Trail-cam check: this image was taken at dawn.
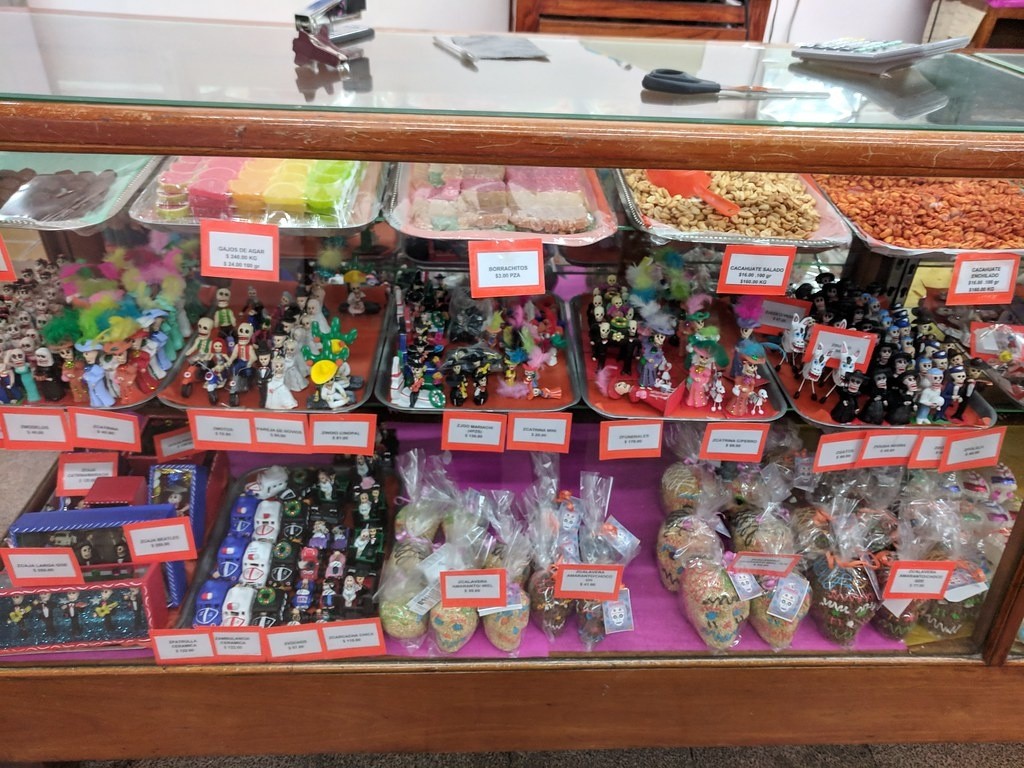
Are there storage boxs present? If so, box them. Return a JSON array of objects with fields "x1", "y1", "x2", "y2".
[
  {"x1": 83, "y1": 477, "x2": 148, "y2": 507},
  {"x1": 2, "y1": 504, "x2": 189, "y2": 612},
  {"x1": 149, "y1": 464, "x2": 206, "y2": 551},
  {"x1": 127, "y1": 414, "x2": 208, "y2": 466},
  {"x1": 0, "y1": 563, "x2": 169, "y2": 647}
]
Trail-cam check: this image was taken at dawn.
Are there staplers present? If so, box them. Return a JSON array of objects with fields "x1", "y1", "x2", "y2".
[{"x1": 291, "y1": 0, "x2": 381, "y2": 49}]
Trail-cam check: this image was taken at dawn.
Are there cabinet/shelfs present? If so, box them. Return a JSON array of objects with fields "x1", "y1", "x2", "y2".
[
  {"x1": 0, "y1": 6, "x2": 1024, "y2": 764},
  {"x1": 922, "y1": 0, "x2": 1024, "y2": 48}
]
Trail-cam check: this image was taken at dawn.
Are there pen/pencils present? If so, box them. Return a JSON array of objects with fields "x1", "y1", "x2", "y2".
[{"x1": 429, "y1": 31, "x2": 478, "y2": 66}]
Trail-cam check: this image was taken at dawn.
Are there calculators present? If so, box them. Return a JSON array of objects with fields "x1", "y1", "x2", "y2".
[{"x1": 791, "y1": 20, "x2": 974, "y2": 77}]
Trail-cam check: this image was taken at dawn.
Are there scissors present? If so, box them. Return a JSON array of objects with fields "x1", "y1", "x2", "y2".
[{"x1": 638, "y1": 61, "x2": 832, "y2": 104}]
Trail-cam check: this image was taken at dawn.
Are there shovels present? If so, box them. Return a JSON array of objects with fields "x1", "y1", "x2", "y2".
[{"x1": 643, "y1": 167, "x2": 744, "y2": 221}]
